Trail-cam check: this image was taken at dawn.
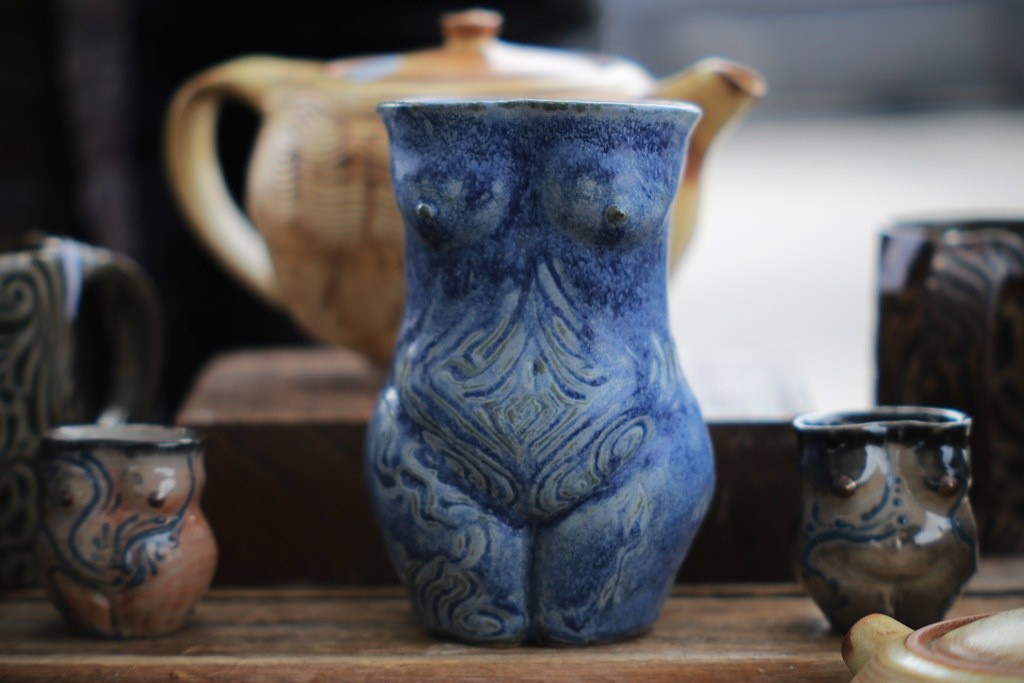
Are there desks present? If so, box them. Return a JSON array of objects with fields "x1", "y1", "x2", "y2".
[{"x1": 0, "y1": 553, "x2": 1024, "y2": 683}]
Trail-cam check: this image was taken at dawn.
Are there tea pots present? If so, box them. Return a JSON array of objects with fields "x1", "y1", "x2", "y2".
[
  {"x1": 838, "y1": 607, "x2": 1024, "y2": 683},
  {"x1": 168, "y1": 10, "x2": 773, "y2": 388}
]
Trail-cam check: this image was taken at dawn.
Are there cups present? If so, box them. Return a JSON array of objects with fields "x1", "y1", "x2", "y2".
[
  {"x1": 0, "y1": 225, "x2": 172, "y2": 593},
  {"x1": 367, "y1": 95, "x2": 718, "y2": 650},
  {"x1": 41, "y1": 422, "x2": 220, "y2": 639},
  {"x1": 792, "y1": 405, "x2": 987, "y2": 640},
  {"x1": 873, "y1": 220, "x2": 1024, "y2": 560}
]
